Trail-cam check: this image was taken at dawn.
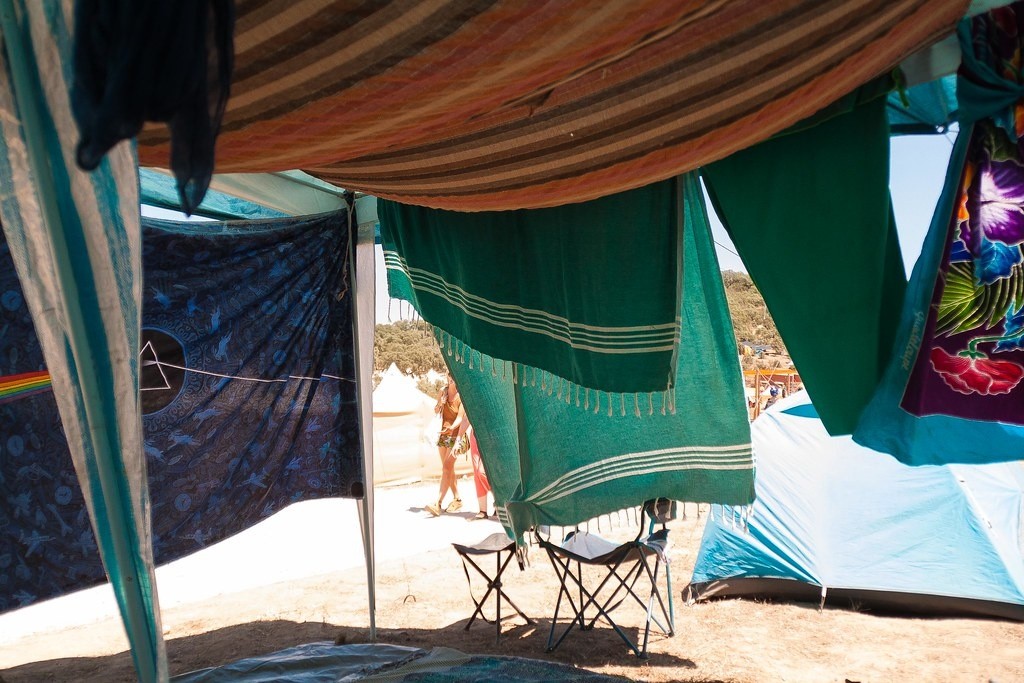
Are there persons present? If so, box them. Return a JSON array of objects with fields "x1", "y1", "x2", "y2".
[
  {"x1": 424, "y1": 372, "x2": 465, "y2": 517},
  {"x1": 451, "y1": 412, "x2": 497, "y2": 518}
]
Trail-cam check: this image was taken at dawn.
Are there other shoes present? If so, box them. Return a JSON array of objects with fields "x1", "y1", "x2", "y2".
[{"x1": 475, "y1": 511, "x2": 488, "y2": 518}]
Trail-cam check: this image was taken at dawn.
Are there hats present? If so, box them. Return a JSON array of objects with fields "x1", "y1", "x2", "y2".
[{"x1": 770, "y1": 388, "x2": 778, "y2": 396}]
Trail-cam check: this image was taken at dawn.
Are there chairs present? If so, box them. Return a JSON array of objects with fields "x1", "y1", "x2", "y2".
[{"x1": 453, "y1": 496, "x2": 678, "y2": 654}]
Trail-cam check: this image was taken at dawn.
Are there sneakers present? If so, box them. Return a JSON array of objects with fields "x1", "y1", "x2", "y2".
[
  {"x1": 425, "y1": 504, "x2": 441, "y2": 515},
  {"x1": 445, "y1": 497, "x2": 463, "y2": 512}
]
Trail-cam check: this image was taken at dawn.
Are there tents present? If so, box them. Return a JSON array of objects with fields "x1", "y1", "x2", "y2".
[{"x1": 682, "y1": 389, "x2": 1024, "y2": 620}]
